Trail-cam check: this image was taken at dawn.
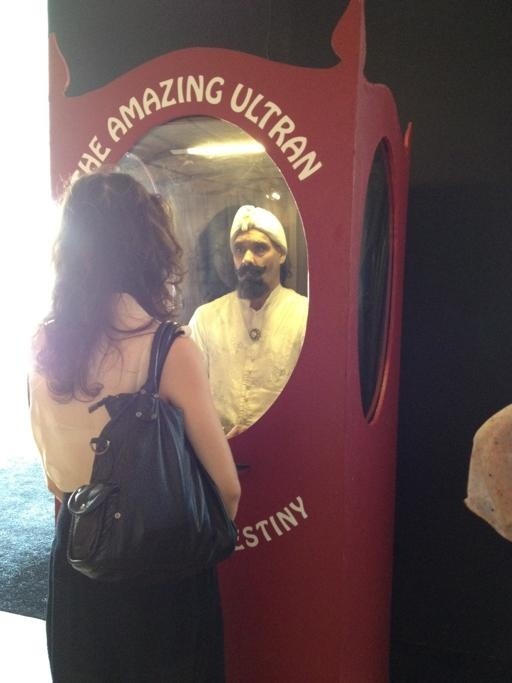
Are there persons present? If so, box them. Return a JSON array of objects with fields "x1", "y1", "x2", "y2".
[
  {"x1": 22, "y1": 167, "x2": 242, "y2": 683},
  {"x1": 185, "y1": 204, "x2": 305, "y2": 438}
]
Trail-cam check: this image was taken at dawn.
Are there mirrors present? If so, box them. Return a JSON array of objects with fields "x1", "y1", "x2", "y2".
[
  {"x1": 111, "y1": 115, "x2": 310, "y2": 439},
  {"x1": 358, "y1": 135, "x2": 399, "y2": 423}
]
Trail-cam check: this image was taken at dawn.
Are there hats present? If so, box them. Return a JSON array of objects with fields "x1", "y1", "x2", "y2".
[{"x1": 223, "y1": 200, "x2": 288, "y2": 257}]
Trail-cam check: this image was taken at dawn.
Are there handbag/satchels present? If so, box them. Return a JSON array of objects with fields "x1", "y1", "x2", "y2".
[{"x1": 60, "y1": 319, "x2": 241, "y2": 585}]
